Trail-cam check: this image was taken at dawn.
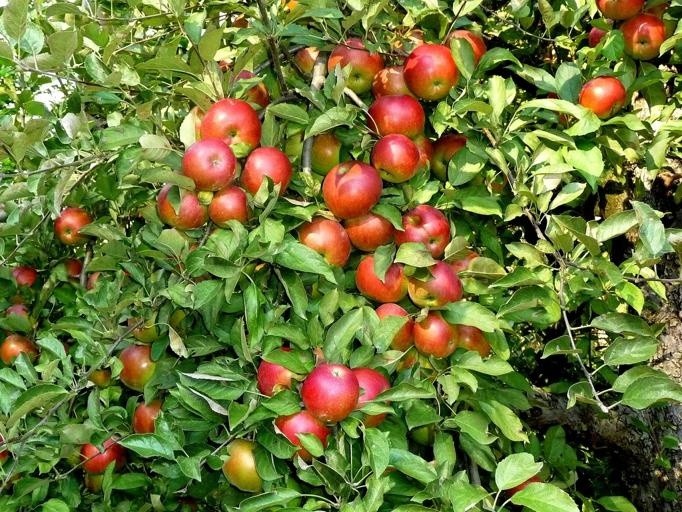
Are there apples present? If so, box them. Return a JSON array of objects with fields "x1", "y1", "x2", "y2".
[{"x1": 1, "y1": 0, "x2": 670, "y2": 493}]
